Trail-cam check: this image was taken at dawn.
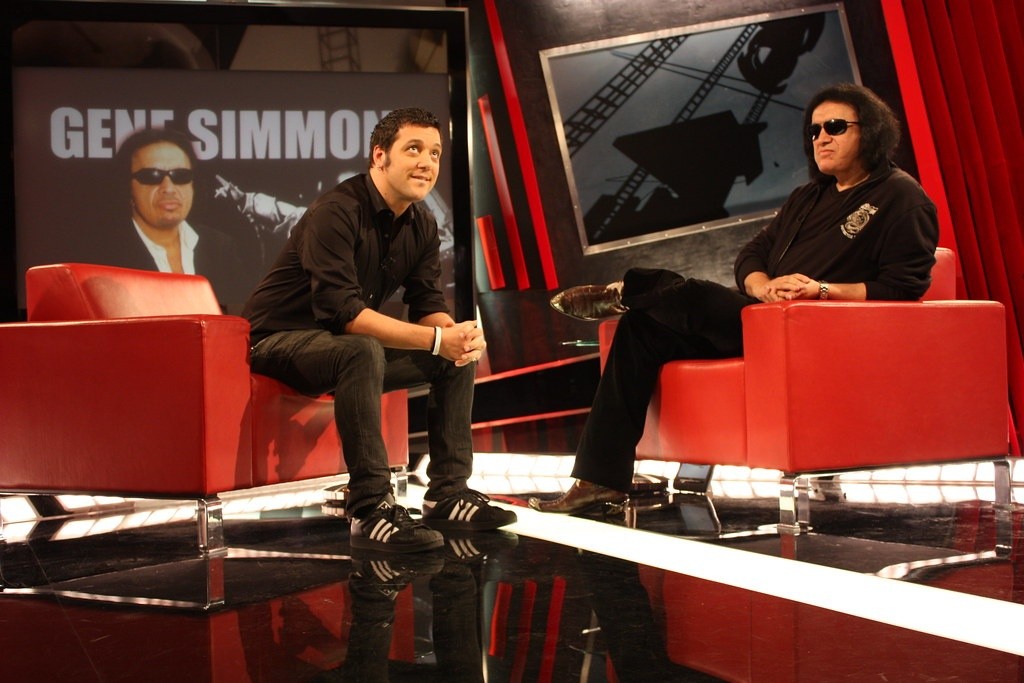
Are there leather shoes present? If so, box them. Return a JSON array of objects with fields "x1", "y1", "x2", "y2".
[
  {"x1": 550, "y1": 282, "x2": 629, "y2": 322},
  {"x1": 527, "y1": 479, "x2": 628, "y2": 516}
]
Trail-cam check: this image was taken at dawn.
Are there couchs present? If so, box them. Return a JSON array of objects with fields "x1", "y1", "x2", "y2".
[
  {"x1": 598, "y1": 245, "x2": 1024, "y2": 534},
  {"x1": 0, "y1": 264, "x2": 409, "y2": 553}
]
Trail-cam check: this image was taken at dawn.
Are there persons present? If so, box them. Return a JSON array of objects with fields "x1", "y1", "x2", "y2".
[
  {"x1": 529, "y1": 84, "x2": 940, "y2": 513},
  {"x1": 244, "y1": 110, "x2": 517, "y2": 552},
  {"x1": 529, "y1": 548, "x2": 669, "y2": 682},
  {"x1": 88, "y1": 129, "x2": 235, "y2": 279},
  {"x1": 345, "y1": 550, "x2": 486, "y2": 683}
]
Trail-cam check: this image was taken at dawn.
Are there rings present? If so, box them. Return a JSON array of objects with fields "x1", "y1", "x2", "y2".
[{"x1": 473, "y1": 359, "x2": 476, "y2": 361}]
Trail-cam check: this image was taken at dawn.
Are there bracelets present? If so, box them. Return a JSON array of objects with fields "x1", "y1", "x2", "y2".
[
  {"x1": 819, "y1": 283, "x2": 829, "y2": 300},
  {"x1": 431, "y1": 326, "x2": 441, "y2": 355}
]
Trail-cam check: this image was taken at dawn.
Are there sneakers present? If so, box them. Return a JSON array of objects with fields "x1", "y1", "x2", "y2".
[
  {"x1": 437, "y1": 529, "x2": 518, "y2": 565},
  {"x1": 421, "y1": 488, "x2": 518, "y2": 530},
  {"x1": 348, "y1": 555, "x2": 442, "y2": 605},
  {"x1": 347, "y1": 491, "x2": 445, "y2": 553}
]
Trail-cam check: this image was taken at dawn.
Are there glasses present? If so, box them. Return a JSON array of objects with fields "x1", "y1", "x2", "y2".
[
  {"x1": 808, "y1": 119, "x2": 860, "y2": 140},
  {"x1": 129, "y1": 167, "x2": 196, "y2": 185}
]
